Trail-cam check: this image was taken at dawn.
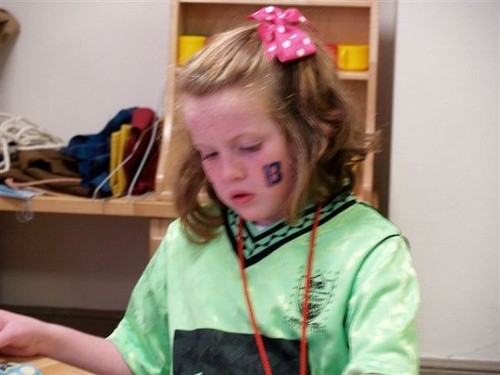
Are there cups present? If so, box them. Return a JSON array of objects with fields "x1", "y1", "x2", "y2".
[
  {"x1": 177, "y1": 35, "x2": 208, "y2": 66},
  {"x1": 336, "y1": 44, "x2": 369, "y2": 70}
]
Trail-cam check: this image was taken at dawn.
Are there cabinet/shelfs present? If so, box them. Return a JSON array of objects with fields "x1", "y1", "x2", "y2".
[{"x1": 0, "y1": 0, "x2": 378, "y2": 266}]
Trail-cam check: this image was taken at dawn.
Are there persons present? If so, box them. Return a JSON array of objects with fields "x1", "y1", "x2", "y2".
[{"x1": 0, "y1": 6, "x2": 420, "y2": 375}]
